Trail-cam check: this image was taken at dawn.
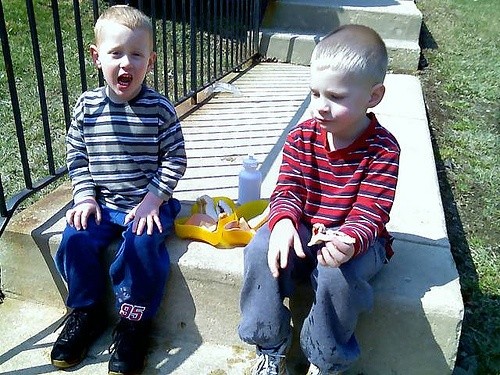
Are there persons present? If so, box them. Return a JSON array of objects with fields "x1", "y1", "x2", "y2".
[
  {"x1": 239, "y1": 24, "x2": 401, "y2": 375},
  {"x1": 50, "y1": 5, "x2": 187, "y2": 375}
]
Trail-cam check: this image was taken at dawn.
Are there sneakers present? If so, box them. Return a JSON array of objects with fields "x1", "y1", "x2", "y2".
[
  {"x1": 250, "y1": 325, "x2": 292, "y2": 375},
  {"x1": 306, "y1": 363, "x2": 344, "y2": 375},
  {"x1": 107, "y1": 317, "x2": 151, "y2": 375},
  {"x1": 51, "y1": 308, "x2": 104, "y2": 367}
]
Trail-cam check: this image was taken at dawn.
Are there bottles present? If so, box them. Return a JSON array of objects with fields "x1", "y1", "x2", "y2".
[{"x1": 238, "y1": 159, "x2": 262, "y2": 206}]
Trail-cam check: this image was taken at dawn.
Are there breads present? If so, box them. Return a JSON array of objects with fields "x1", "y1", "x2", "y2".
[
  {"x1": 223, "y1": 206, "x2": 270, "y2": 233},
  {"x1": 184, "y1": 194, "x2": 234, "y2": 232}
]
